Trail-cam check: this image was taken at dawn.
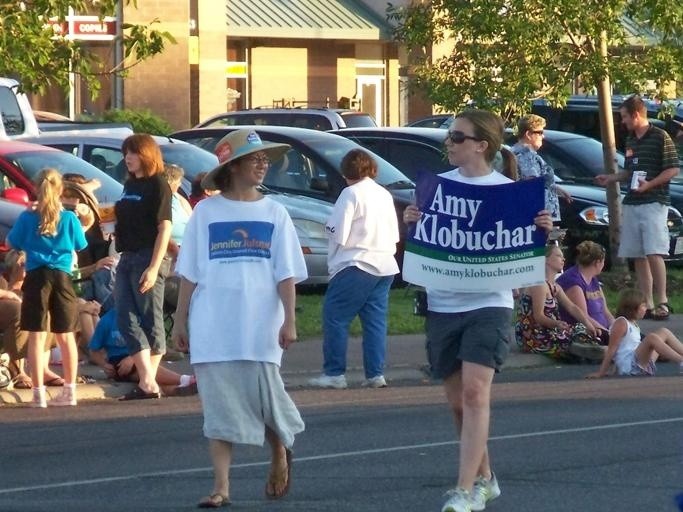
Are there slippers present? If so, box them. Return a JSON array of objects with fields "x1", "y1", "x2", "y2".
[
  {"x1": 118, "y1": 386, "x2": 160, "y2": 401},
  {"x1": 264, "y1": 449, "x2": 291, "y2": 500},
  {"x1": 14, "y1": 380, "x2": 32, "y2": 389},
  {"x1": 44, "y1": 378, "x2": 65, "y2": 387},
  {"x1": 198, "y1": 493, "x2": 232, "y2": 507}
]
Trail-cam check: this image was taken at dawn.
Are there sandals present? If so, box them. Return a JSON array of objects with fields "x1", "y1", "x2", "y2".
[
  {"x1": 654, "y1": 303, "x2": 673, "y2": 321},
  {"x1": 642, "y1": 307, "x2": 654, "y2": 320}
]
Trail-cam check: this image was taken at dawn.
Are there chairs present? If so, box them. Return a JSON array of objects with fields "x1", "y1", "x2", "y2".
[
  {"x1": 263, "y1": 154, "x2": 298, "y2": 191},
  {"x1": 115, "y1": 158, "x2": 130, "y2": 185},
  {"x1": 91, "y1": 155, "x2": 106, "y2": 171}
]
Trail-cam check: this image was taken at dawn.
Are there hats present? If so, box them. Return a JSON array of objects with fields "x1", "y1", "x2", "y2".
[{"x1": 199, "y1": 128, "x2": 291, "y2": 190}]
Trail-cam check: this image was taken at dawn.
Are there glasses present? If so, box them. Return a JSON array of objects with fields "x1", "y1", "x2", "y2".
[
  {"x1": 241, "y1": 152, "x2": 272, "y2": 163},
  {"x1": 448, "y1": 130, "x2": 480, "y2": 143},
  {"x1": 531, "y1": 130, "x2": 542, "y2": 134}
]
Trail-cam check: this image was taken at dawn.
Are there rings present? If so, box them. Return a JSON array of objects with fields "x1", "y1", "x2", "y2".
[{"x1": 405, "y1": 213, "x2": 409, "y2": 220}]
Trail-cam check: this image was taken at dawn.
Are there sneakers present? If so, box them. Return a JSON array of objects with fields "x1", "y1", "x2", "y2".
[
  {"x1": 309, "y1": 373, "x2": 347, "y2": 389},
  {"x1": 568, "y1": 343, "x2": 608, "y2": 360},
  {"x1": 361, "y1": 375, "x2": 387, "y2": 388},
  {"x1": 471, "y1": 471, "x2": 502, "y2": 511},
  {"x1": 441, "y1": 486, "x2": 471, "y2": 512},
  {"x1": 173, "y1": 383, "x2": 197, "y2": 396},
  {"x1": 29, "y1": 388, "x2": 47, "y2": 408},
  {"x1": 46, "y1": 387, "x2": 77, "y2": 406}
]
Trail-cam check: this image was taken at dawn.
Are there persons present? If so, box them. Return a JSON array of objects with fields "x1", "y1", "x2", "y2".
[
  {"x1": 514, "y1": 245, "x2": 614, "y2": 364},
  {"x1": 587, "y1": 290, "x2": 682, "y2": 377},
  {"x1": 557, "y1": 241, "x2": 646, "y2": 345},
  {"x1": 399, "y1": 110, "x2": 555, "y2": 512},
  {"x1": 172, "y1": 130, "x2": 306, "y2": 508},
  {"x1": 505, "y1": 114, "x2": 573, "y2": 280},
  {"x1": 308, "y1": 150, "x2": 400, "y2": 389},
  {"x1": 1, "y1": 133, "x2": 221, "y2": 409},
  {"x1": 595, "y1": 97, "x2": 680, "y2": 319}
]
